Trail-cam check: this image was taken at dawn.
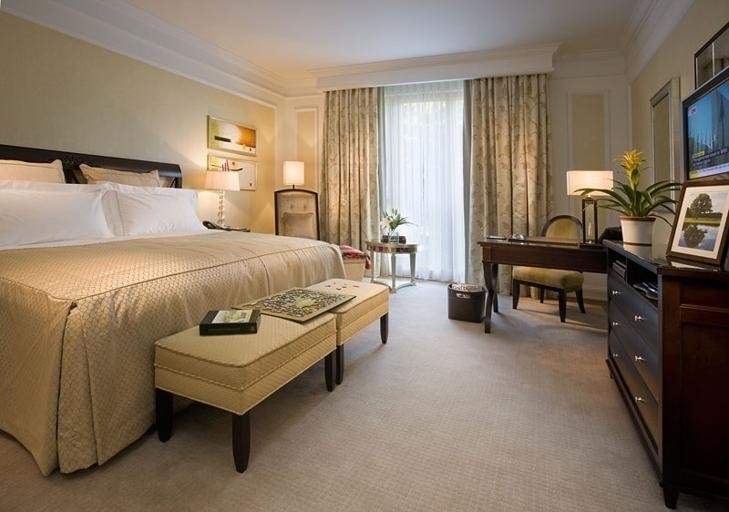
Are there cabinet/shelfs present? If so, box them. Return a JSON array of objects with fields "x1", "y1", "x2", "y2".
[{"x1": 597, "y1": 234, "x2": 728, "y2": 510}]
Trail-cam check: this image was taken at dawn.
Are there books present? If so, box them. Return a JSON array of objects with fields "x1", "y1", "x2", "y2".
[
  {"x1": 198, "y1": 309, "x2": 261, "y2": 334},
  {"x1": 381, "y1": 235, "x2": 406, "y2": 244}
]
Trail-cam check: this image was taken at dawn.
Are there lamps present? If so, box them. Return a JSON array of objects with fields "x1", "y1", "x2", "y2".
[
  {"x1": 564, "y1": 168, "x2": 618, "y2": 249},
  {"x1": 281, "y1": 158, "x2": 305, "y2": 189},
  {"x1": 201, "y1": 169, "x2": 242, "y2": 230}
]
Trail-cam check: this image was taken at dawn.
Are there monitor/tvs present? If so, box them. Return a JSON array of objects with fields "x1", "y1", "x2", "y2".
[{"x1": 680, "y1": 64, "x2": 729, "y2": 180}]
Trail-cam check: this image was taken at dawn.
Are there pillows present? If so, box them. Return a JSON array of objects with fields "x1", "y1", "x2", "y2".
[
  {"x1": 0, "y1": 156, "x2": 66, "y2": 185},
  {"x1": 0, "y1": 176, "x2": 118, "y2": 255},
  {"x1": 279, "y1": 210, "x2": 316, "y2": 240},
  {"x1": 75, "y1": 160, "x2": 163, "y2": 189},
  {"x1": 91, "y1": 179, "x2": 209, "y2": 240}
]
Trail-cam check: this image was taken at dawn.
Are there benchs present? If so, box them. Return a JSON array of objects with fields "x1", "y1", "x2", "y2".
[
  {"x1": 299, "y1": 274, "x2": 392, "y2": 388},
  {"x1": 150, "y1": 297, "x2": 340, "y2": 476}
]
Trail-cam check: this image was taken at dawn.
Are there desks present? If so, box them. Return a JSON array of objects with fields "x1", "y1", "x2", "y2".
[
  {"x1": 474, "y1": 232, "x2": 609, "y2": 336},
  {"x1": 362, "y1": 236, "x2": 420, "y2": 296}
]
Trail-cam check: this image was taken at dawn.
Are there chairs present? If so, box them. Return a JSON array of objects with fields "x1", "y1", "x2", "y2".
[
  {"x1": 271, "y1": 188, "x2": 322, "y2": 243},
  {"x1": 511, "y1": 213, "x2": 590, "y2": 324}
]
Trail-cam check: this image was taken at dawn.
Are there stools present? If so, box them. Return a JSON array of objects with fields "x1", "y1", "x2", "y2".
[{"x1": 341, "y1": 250, "x2": 369, "y2": 282}]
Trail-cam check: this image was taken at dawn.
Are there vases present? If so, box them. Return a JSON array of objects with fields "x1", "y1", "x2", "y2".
[
  {"x1": 616, "y1": 214, "x2": 656, "y2": 248},
  {"x1": 386, "y1": 229, "x2": 402, "y2": 246}
]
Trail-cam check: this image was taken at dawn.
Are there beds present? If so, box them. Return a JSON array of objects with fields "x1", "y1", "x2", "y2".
[{"x1": 0, "y1": 141, "x2": 351, "y2": 479}]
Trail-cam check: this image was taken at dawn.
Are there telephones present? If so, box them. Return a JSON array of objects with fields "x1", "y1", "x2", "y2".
[{"x1": 203, "y1": 221, "x2": 223, "y2": 229}]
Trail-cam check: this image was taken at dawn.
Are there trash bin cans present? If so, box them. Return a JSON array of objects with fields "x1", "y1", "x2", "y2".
[{"x1": 447, "y1": 281, "x2": 487, "y2": 323}]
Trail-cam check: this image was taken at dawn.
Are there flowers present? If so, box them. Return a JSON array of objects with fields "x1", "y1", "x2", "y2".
[
  {"x1": 377, "y1": 206, "x2": 421, "y2": 233},
  {"x1": 568, "y1": 145, "x2": 683, "y2": 230}
]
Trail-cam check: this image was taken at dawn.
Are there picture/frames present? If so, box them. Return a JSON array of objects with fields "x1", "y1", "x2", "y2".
[
  {"x1": 206, "y1": 151, "x2": 259, "y2": 192},
  {"x1": 662, "y1": 178, "x2": 728, "y2": 269},
  {"x1": 206, "y1": 114, "x2": 257, "y2": 159}
]
